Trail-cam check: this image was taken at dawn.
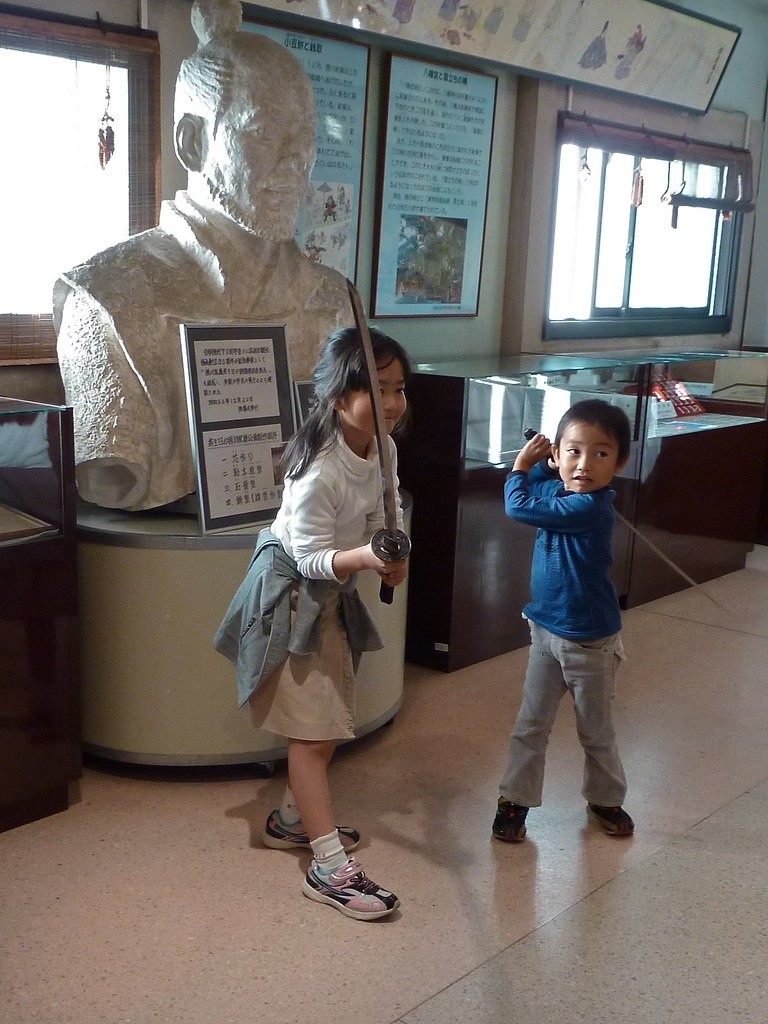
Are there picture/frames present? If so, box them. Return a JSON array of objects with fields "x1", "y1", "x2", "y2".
[
  {"x1": 239, "y1": 0, "x2": 742, "y2": 115},
  {"x1": 369, "y1": 51, "x2": 499, "y2": 320},
  {"x1": 240, "y1": 16, "x2": 371, "y2": 287}
]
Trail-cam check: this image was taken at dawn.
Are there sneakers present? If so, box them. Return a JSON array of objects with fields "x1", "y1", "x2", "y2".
[
  {"x1": 492, "y1": 796, "x2": 530, "y2": 841},
  {"x1": 262, "y1": 809, "x2": 361, "y2": 852},
  {"x1": 586, "y1": 801, "x2": 635, "y2": 835},
  {"x1": 302, "y1": 860, "x2": 400, "y2": 921}
]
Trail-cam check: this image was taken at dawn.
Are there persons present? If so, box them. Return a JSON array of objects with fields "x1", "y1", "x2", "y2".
[
  {"x1": 261, "y1": 327, "x2": 410, "y2": 921},
  {"x1": 52, "y1": 0, "x2": 360, "y2": 514},
  {"x1": 485, "y1": 399, "x2": 635, "y2": 842}
]
